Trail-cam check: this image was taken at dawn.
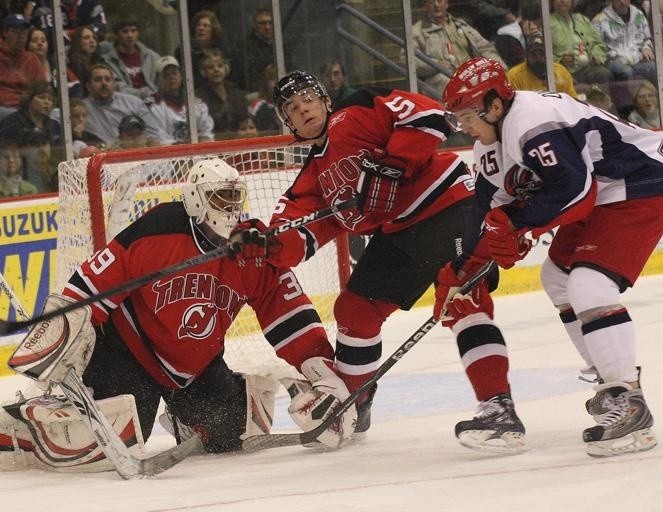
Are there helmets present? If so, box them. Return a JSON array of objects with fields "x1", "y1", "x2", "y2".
[
  {"x1": 274, "y1": 69, "x2": 328, "y2": 126},
  {"x1": 442, "y1": 56, "x2": 514, "y2": 134},
  {"x1": 181, "y1": 157, "x2": 246, "y2": 247}
]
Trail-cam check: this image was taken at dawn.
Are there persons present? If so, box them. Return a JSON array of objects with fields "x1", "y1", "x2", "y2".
[
  {"x1": 0, "y1": 3, "x2": 661, "y2": 197},
  {"x1": 432, "y1": 57, "x2": 663, "y2": 443},
  {"x1": 227, "y1": 69, "x2": 526, "y2": 443},
  {"x1": 0, "y1": 158, "x2": 359, "y2": 473}
]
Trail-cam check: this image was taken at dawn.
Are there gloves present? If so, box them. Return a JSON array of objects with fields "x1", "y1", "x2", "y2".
[
  {"x1": 484, "y1": 207, "x2": 531, "y2": 269},
  {"x1": 434, "y1": 261, "x2": 482, "y2": 328},
  {"x1": 225, "y1": 217, "x2": 282, "y2": 269},
  {"x1": 357, "y1": 155, "x2": 404, "y2": 214}
]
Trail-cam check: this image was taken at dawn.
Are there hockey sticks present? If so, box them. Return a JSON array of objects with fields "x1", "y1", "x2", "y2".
[
  {"x1": 243, "y1": 261, "x2": 497, "y2": 453},
  {"x1": 0, "y1": 271, "x2": 202, "y2": 481}
]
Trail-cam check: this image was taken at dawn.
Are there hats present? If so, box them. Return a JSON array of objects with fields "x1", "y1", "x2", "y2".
[
  {"x1": 2, "y1": 13, "x2": 30, "y2": 31},
  {"x1": 119, "y1": 115, "x2": 145, "y2": 132},
  {"x1": 526, "y1": 35, "x2": 543, "y2": 54},
  {"x1": 154, "y1": 56, "x2": 180, "y2": 74}
]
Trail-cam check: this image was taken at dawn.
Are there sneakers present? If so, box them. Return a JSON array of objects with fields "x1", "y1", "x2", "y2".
[
  {"x1": 456, "y1": 384, "x2": 524, "y2": 442},
  {"x1": 586, "y1": 382, "x2": 612, "y2": 417},
  {"x1": 583, "y1": 389, "x2": 653, "y2": 442},
  {"x1": 158, "y1": 410, "x2": 196, "y2": 442},
  {"x1": 350, "y1": 384, "x2": 378, "y2": 434}
]
